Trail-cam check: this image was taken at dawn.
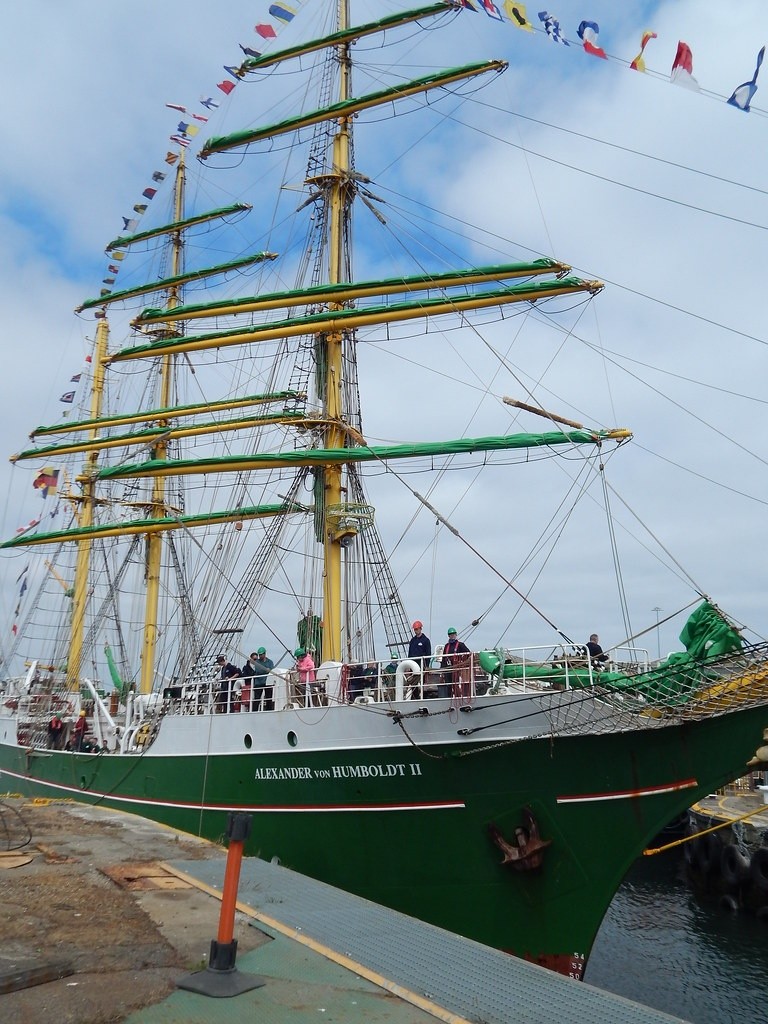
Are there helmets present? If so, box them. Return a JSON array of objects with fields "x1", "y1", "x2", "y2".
[
  {"x1": 294, "y1": 649, "x2": 305, "y2": 657},
  {"x1": 392, "y1": 654, "x2": 398, "y2": 659},
  {"x1": 56, "y1": 712, "x2": 62, "y2": 718},
  {"x1": 79, "y1": 711, "x2": 86, "y2": 717},
  {"x1": 447, "y1": 628, "x2": 457, "y2": 633},
  {"x1": 257, "y1": 647, "x2": 266, "y2": 654},
  {"x1": 413, "y1": 622, "x2": 422, "y2": 630}
]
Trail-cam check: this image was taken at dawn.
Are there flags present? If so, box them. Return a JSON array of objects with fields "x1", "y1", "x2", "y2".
[
  {"x1": 11, "y1": 355, "x2": 91, "y2": 634},
  {"x1": 458, "y1": 0, "x2": 765, "y2": 112},
  {"x1": 95, "y1": 1, "x2": 299, "y2": 318}
]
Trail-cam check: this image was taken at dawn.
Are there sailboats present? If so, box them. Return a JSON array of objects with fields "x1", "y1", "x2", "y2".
[{"x1": 0, "y1": 0, "x2": 768, "y2": 990}]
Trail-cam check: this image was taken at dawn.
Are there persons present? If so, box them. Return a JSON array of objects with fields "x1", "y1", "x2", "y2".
[
  {"x1": 348, "y1": 660, "x2": 378, "y2": 702},
  {"x1": 382, "y1": 654, "x2": 398, "y2": 700},
  {"x1": 294, "y1": 649, "x2": 316, "y2": 706},
  {"x1": 217, "y1": 657, "x2": 241, "y2": 712},
  {"x1": 583, "y1": 634, "x2": 609, "y2": 663},
  {"x1": 251, "y1": 648, "x2": 274, "y2": 711},
  {"x1": 65, "y1": 711, "x2": 110, "y2": 753},
  {"x1": 47, "y1": 713, "x2": 63, "y2": 750},
  {"x1": 440, "y1": 628, "x2": 470, "y2": 683},
  {"x1": 242, "y1": 653, "x2": 258, "y2": 684},
  {"x1": 408, "y1": 621, "x2": 431, "y2": 699}
]
